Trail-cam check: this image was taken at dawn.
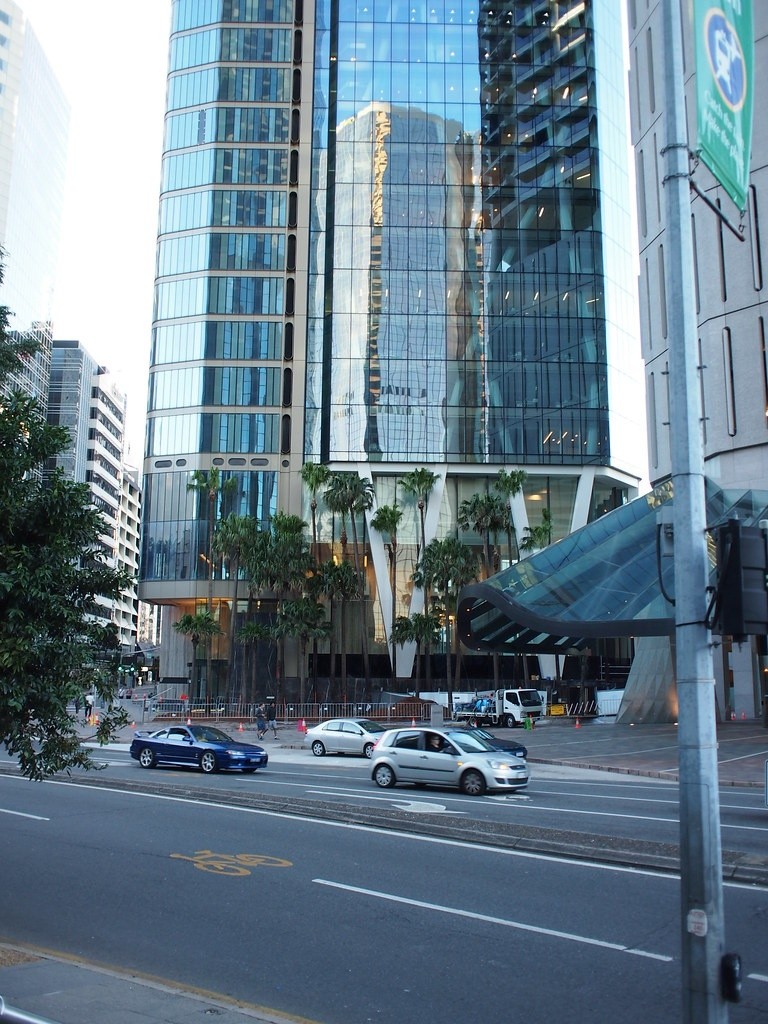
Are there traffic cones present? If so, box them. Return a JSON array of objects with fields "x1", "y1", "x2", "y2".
[
  {"x1": 301, "y1": 716, "x2": 307, "y2": 732},
  {"x1": 239, "y1": 721, "x2": 243, "y2": 731},
  {"x1": 187, "y1": 716, "x2": 192, "y2": 724},
  {"x1": 411, "y1": 717, "x2": 417, "y2": 727}
]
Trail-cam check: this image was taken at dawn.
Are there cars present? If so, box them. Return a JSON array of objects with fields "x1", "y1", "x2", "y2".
[
  {"x1": 304, "y1": 718, "x2": 388, "y2": 758},
  {"x1": 463, "y1": 728, "x2": 529, "y2": 761},
  {"x1": 130, "y1": 724, "x2": 268, "y2": 774},
  {"x1": 368, "y1": 726, "x2": 529, "y2": 795}
]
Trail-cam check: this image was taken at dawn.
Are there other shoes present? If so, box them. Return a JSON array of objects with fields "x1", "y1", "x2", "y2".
[
  {"x1": 274, "y1": 736, "x2": 280, "y2": 740},
  {"x1": 257, "y1": 734, "x2": 264, "y2": 740}
]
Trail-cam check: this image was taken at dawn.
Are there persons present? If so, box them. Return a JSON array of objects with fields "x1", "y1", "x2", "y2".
[
  {"x1": 118, "y1": 687, "x2": 125, "y2": 699},
  {"x1": 148, "y1": 692, "x2": 154, "y2": 699},
  {"x1": 261, "y1": 700, "x2": 279, "y2": 740},
  {"x1": 256, "y1": 701, "x2": 266, "y2": 739},
  {"x1": 74, "y1": 698, "x2": 80, "y2": 718},
  {"x1": 126, "y1": 688, "x2": 132, "y2": 699},
  {"x1": 84, "y1": 692, "x2": 94, "y2": 718}
]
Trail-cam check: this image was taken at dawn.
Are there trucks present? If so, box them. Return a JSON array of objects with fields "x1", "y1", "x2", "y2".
[{"x1": 452, "y1": 688, "x2": 544, "y2": 728}]
